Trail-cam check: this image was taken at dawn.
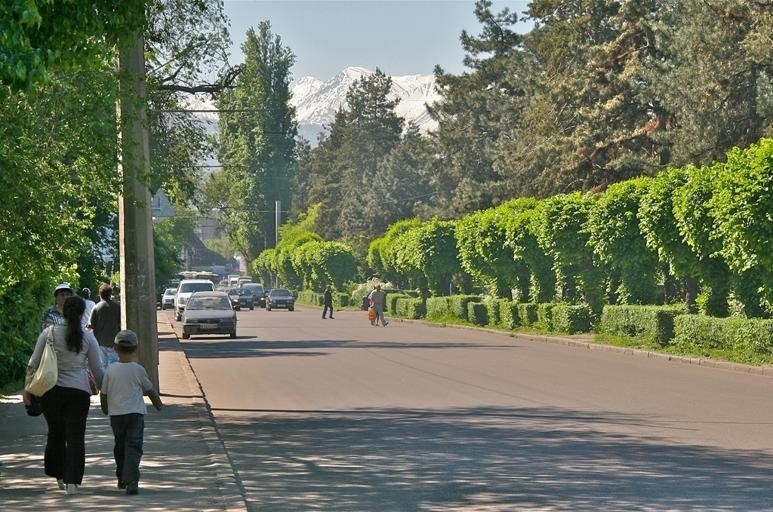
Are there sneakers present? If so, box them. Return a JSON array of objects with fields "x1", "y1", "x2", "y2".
[
  {"x1": 118, "y1": 478, "x2": 126, "y2": 489},
  {"x1": 67, "y1": 485, "x2": 79, "y2": 495},
  {"x1": 322, "y1": 316, "x2": 335, "y2": 320},
  {"x1": 372, "y1": 322, "x2": 389, "y2": 327},
  {"x1": 125, "y1": 482, "x2": 138, "y2": 494},
  {"x1": 57, "y1": 479, "x2": 66, "y2": 490}
]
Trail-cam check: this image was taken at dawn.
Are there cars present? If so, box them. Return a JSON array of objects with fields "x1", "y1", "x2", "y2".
[
  {"x1": 161, "y1": 264, "x2": 293, "y2": 320},
  {"x1": 179, "y1": 290, "x2": 240, "y2": 339}
]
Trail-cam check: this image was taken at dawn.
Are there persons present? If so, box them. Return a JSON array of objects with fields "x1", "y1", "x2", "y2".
[
  {"x1": 322, "y1": 285, "x2": 334, "y2": 319},
  {"x1": 21, "y1": 296, "x2": 104, "y2": 496},
  {"x1": 100, "y1": 329, "x2": 163, "y2": 497},
  {"x1": 366, "y1": 284, "x2": 389, "y2": 328},
  {"x1": 39, "y1": 284, "x2": 74, "y2": 332},
  {"x1": 85, "y1": 284, "x2": 120, "y2": 373},
  {"x1": 80, "y1": 287, "x2": 96, "y2": 330}
]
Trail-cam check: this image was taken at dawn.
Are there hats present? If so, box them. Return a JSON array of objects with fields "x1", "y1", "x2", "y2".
[
  {"x1": 54, "y1": 280, "x2": 73, "y2": 296},
  {"x1": 114, "y1": 329, "x2": 138, "y2": 347}
]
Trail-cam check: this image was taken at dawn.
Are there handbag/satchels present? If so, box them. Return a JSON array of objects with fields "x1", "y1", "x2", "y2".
[
  {"x1": 24, "y1": 323, "x2": 59, "y2": 397},
  {"x1": 25, "y1": 388, "x2": 45, "y2": 418}
]
